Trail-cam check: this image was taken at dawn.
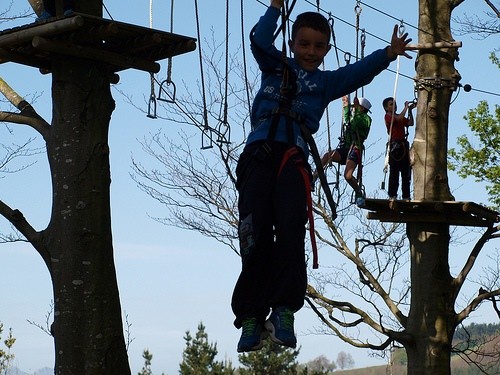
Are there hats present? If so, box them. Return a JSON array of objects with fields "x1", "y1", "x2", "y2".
[{"x1": 349, "y1": 98, "x2": 373, "y2": 114}]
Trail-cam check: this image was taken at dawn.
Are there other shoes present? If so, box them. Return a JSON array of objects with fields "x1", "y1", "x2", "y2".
[
  {"x1": 310, "y1": 182, "x2": 315, "y2": 192},
  {"x1": 357, "y1": 192, "x2": 365, "y2": 207}
]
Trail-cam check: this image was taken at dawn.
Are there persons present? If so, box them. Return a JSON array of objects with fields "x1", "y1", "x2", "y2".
[
  {"x1": 310, "y1": 94, "x2": 373, "y2": 206},
  {"x1": 382, "y1": 97, "x2": 417, "y2": 201},
  {"x1": 231, "y1": 0, "x2": 413, "y2": 352}
]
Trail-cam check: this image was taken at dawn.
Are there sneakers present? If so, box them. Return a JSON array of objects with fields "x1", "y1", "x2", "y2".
[
  {"x1": 237, "y1": 317, "x2": 268, "y2": 353},
  {"x1": 266, "y1": 309, "x2": 297, "y2": 349}
]
